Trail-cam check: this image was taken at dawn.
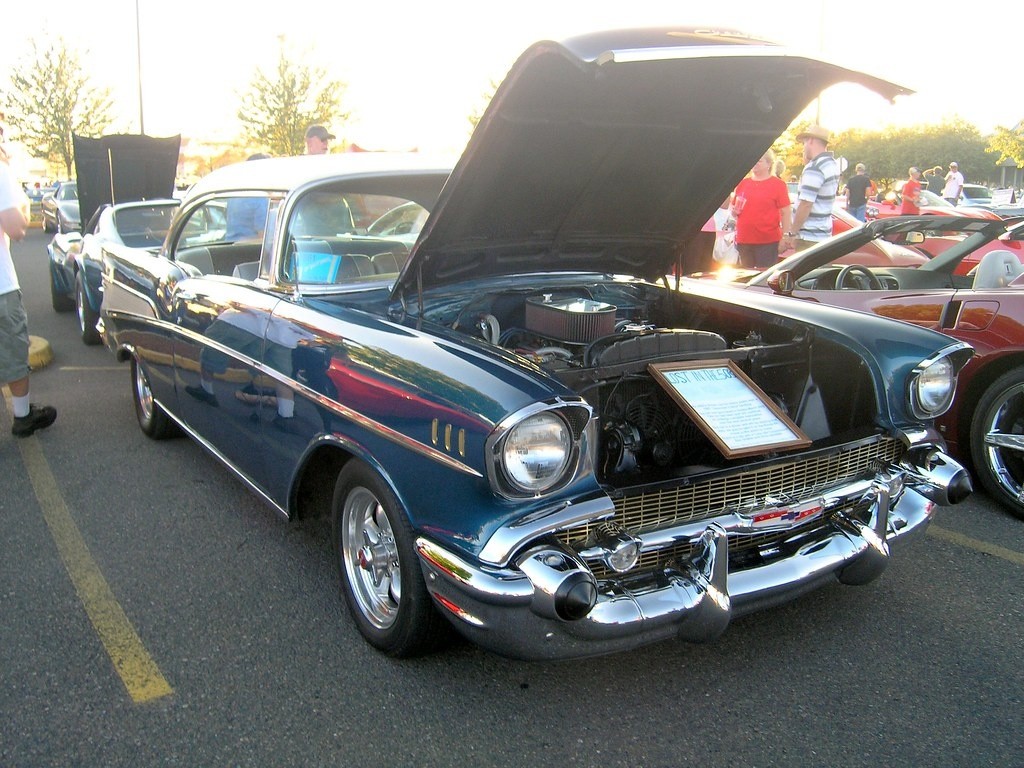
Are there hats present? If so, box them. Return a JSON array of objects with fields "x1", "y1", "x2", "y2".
[
  {"x1": 856, "y1": 163, "x2": 865, "y2": 171},
  {"x1": 909, "y1": 167, "x2": 923, "y2": 174},
  {"x1": 307, "y1": 126, "x2": 336, "y2": 141},
  {"x1": 796, "y1": 126, "x2": 830, "y2": 144}
]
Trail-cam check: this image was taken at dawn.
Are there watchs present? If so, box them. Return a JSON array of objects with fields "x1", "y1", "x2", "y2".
[{"x1": 788, "y1": 231, "x2": 797, "y2": 237}]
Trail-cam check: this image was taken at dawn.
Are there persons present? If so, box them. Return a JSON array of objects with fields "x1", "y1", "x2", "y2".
[
  {"x1": 0, "y1": 112, "x2": 56, "y2": 437},
  {"x1": 900, "y1": 167, "x2": 922, "y2": 216},
  {"x1": 789, "y1": 124, "x2": 840, "y2": 255},
  {"x1": 222, "y1": 153, "x2": 272, "y2": 242},
  {"x1": 842, "y1": 163, "x2": 878, "y2": 222},
  {"x1": 731, "y1": 148, "x2": 791, "y2": 267},
  {"x1": 290, "y1": 125, "x2": 355, "y2": 236},
  {"x1": 681, "y1": 193, "x2": 730, "y2": 272},
  {"x1": 921, "y1": 165, "x2": 946, "y2": 196},
  {"x1": 944, "y1": 161, "x2": 964, "y2": 207},
  {"x1": 182, "y1": 297, "x2": 338, "y2": 437}
]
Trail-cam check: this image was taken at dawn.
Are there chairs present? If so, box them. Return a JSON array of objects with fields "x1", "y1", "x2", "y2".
[
  {"x1": 232, "y1": 251, "x2": 413, "y2": 281},
  {"x1": 973, "y1": 250, "x2": 1023, "y2": 290}
]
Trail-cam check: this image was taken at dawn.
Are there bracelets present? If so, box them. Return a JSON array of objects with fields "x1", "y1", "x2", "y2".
[
  {"x1": 783, "y1": 233, "x2": 789, "y2": 236},
  {"x1": 782, "y1": 237, "x2": 789, "y2": 239}
]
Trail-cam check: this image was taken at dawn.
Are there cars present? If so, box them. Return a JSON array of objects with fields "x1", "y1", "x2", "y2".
[
  {"x1": 95, "y1": 27, "x2": 976, "y2": 664},
  {"x1": 709, "y1": 182, "x2": 1023, "y2": 276},
  {"x1": 24, "y1": 132, "x2": 431, "y2": 347}
]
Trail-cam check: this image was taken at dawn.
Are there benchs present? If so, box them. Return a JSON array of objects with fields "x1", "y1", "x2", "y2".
[{"x1": 177, "y1": 239, "x2": 415, "y2": 276}]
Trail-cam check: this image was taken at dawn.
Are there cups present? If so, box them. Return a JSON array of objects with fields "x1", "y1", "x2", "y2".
[{"x1": 735, "y1": 196, "x2": 746, "y2": 215}]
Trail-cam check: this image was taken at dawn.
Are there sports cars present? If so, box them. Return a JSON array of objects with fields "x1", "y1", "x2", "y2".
[{"x1": 680, "y1": 214, "x2": 1023, "y2": 522}]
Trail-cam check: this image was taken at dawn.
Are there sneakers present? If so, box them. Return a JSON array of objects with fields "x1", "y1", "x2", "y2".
[{"x1": 11, "y1": 404, "x2": 57, "y2": 439}]
